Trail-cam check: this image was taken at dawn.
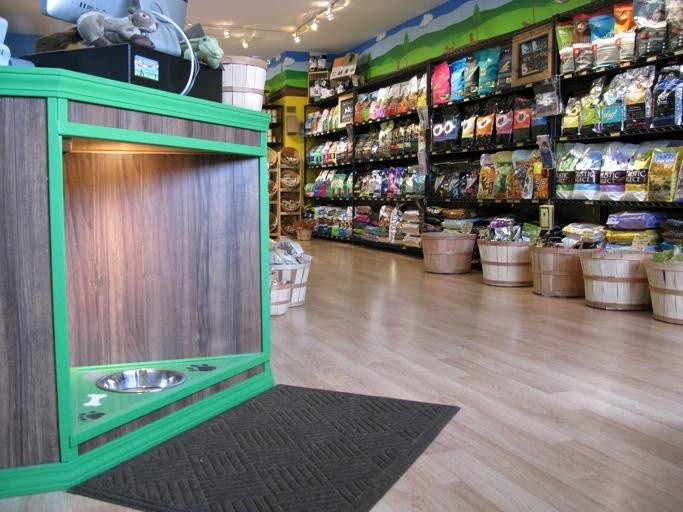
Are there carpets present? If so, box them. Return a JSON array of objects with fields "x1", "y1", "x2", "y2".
[{"x1": 66, "y1": 384, "x2": 461, "y2": 512}]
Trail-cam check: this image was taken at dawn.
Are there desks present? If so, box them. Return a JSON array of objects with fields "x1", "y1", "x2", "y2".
[{"x1": 1, "y1": 66, "x2": 274, "y2": 499}]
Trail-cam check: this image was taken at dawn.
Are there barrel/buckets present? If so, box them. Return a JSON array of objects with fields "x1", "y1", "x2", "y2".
[
  {"x1": 220, "y1": 56, "x2": 268, "y2": 112},
  {"x1": 641, "y1": 257, "x2": 683, "y2": 326},
  {"x1": 296, "y1": 228, "x2": 312, "y2": 241},
  {"x1": 576, "y1": 248, "x2": 654, "y2": 311},
  {"x1": 476, "y1": 238, "x2": 532, "y2": 286},
  {"x1": 529, "y1": 242, "x2": 597, "y2": 297},
  {"x1": 269, "y1": 254, "x2": 313, "y2": 307},
  {"x1": 270, "y1": 280, "x2": 293, "y2": 315},
  {"x1": 421, "y1": 231, "x2": 475, "y2": 274}
]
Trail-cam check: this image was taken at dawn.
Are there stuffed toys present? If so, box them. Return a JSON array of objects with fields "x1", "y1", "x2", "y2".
[
  {"x1": 33, "y1": 5, "x2": 159, "y2": 54},
  {"x1": 177, "y1": 34, "x2": 223, "y2": 70}
]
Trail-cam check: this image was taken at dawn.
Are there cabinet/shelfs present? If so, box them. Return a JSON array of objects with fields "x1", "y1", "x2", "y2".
[
  {"x1": 352, "y1": 65, "x2": 427, "y2": 256},
  {"x1": 303, "y1": 90, "x2": 353, "y2": 244},
  {"x1": 554, "y1": 1, "x2": 682, "y2": 259},
  {"x1": 263, "y1": 103, "x2": 301, "y2": 238},
  {"x1": 425, "y1": 19, "x2": 553, "y2": 245}
]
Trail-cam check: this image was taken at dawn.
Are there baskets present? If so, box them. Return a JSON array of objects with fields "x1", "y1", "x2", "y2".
[{"x1": 268, "y1": 146, "x2": 300, "y2": 236}]
